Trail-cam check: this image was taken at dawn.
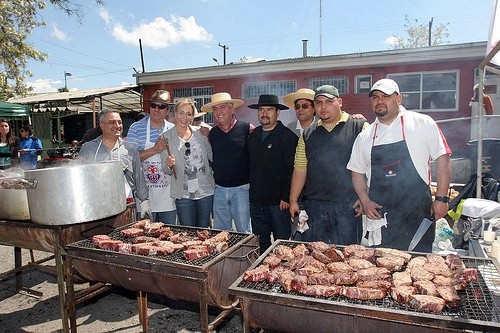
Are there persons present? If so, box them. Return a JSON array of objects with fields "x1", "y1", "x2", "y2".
[
  {"x1": 471, "y1": 83, "x2": 494, "y2": 143},
  {"x1": 165, "y1": 105, "x2": 176, "y2": 124},
  {"x1": 0, "y1": 117, "x2": 19, "y2": 170},
  {"x1": 283, "y1": 88, "x2": 365, "y2": 137},
  {"x1": 126, "y1": 89, "x2": 211, "y2": 224},
  {"x1": 191, "y1": 106, "x2": 213, "y2": 131},
  {"x1": 157, "y1": 95, "x2": 216, "y2": 231},
  {"x1": 19, "y1": 126, "x2": 43, "y2": 168},
  {"x1": 199, "y1": 93, "x2": 257, "y2": 233},
  {"x1": 346, "y1": 77, "x2": 453, "y2": 254},
  {"x1": 76, "y1": 108, "x2": 154, "y2": 223},
  {"x1": 248, "y1": 95, "x2": 300, "y2": 255},
  {"x1": 289, "y1": 84, "x2": 370, "y2": 247}
]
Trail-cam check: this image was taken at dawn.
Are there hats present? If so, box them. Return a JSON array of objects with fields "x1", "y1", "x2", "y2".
[
  {"x1": 194, "y1": 107, "x2": 206, "y2": 118},
  {"x1": 143, "y1": 89, "x2": 176, "y2": 105},
  {"x1": 283, "y1": 88, "x2": 316, "y2": 108},
  {"x1": 369, "y1": 78, "x2": 401, "y2": 96},
  {"x1": 200, "y1": 92, "x2": 245, "y2": 113},
  {"x1": 472, "y1": 83, "x2": 485, "y2": 90},
  {"x1": 247, "y1": 94, "x2": 289, "y2": 110},
  {"x1": 315, "y1": 84, "x2": 339, "y2": 100}
]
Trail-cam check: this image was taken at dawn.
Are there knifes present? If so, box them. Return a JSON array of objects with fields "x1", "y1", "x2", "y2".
[{"x1": 407, "y1": 213, "x2": 436, "y2": 253}]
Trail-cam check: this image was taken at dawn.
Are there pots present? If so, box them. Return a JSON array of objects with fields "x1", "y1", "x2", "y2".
[
  {"x1": 14, "y1": 162, "x2": 128, "y2": 226},
  {"x1": 0, "y1": 175, "x2": 31, "y2": 221}
]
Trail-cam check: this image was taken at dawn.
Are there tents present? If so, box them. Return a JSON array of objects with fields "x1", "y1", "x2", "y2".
[
  {"x1": 9, "y1": 85, "x2": 145, "y2": 131},
  {"x1": 1, "y1": 101, "x2": 31, "y2": 130}
]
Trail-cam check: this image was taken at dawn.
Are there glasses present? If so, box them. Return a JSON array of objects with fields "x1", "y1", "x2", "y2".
[
  {"x1": 185, "y1": 142, "x2": 190, "y2": 156},
  {"x1": 149, "y1": 102, "x2": 167, "y2": 109},
  {"x1": 20, "y1": 129, "x2": 25, "y2": 132},
  {"x1": 293, "y1": 103, "x2": 312, "y2": 109}
]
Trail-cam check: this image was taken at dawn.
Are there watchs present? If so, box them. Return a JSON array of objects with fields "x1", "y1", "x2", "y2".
[{"x1": 435, "y1": 195, "x2": 450, "y2": 203}]
